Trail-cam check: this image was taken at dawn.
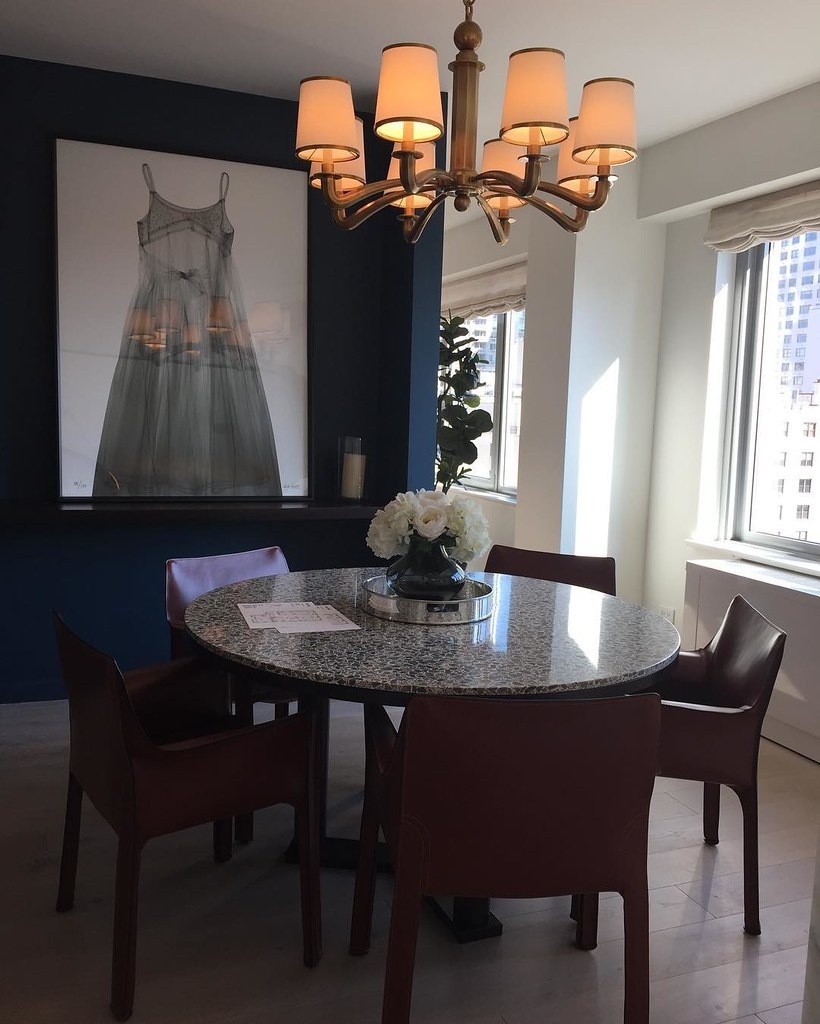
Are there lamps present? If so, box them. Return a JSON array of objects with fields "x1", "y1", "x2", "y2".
[
  {"x1": 293, "y1": 0, "x2": 640, "y2": 245},
  {"x1": 126, "y1": 272, "x2": 232, "y2": 367}
]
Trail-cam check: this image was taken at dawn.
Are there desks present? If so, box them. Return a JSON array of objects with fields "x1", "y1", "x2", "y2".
[
  {"x1": 184, "y1": 567, "x2": 682, "y2": 943},
  {"x1": 0, "y1": 497, "x2": 391, "y2": 531}
]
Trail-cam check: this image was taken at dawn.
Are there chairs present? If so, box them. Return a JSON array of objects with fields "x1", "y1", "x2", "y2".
[
  {"x1": 165, "y1": 546, "x2": 297, "y2": 845},
  {"x1": 53, "y1": 612, "x2": 322, "y2": 1024},
  {"x1": 568, "y1": 594, "x2": 787, "y2": 935},
  {"x1": 482, "y1": 544, "x2": 615, "y2": 598},
  {"x1": 351, "y1": 691, "x2": 662, "y2": 1024}
]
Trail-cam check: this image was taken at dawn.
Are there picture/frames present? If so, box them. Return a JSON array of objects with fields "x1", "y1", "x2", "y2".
[{"x1": 51, "y1": 135, "x2": 318, "y2": 503}]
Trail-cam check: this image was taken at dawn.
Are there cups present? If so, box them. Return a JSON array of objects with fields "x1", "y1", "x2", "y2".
[{"x1": 340, "y1": 451, "x2": 368, "y2": 504}]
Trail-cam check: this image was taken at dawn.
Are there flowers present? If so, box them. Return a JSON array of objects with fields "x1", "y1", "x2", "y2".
[{"x1": 364, "y1": 488, "x2": 492, "y2": 564}]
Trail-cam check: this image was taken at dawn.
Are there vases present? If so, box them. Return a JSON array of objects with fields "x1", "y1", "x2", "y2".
[{"x1": 386, "y1": 539, "x2": 467, "y2": 600}]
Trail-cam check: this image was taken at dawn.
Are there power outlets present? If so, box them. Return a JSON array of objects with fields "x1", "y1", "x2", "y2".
[{"x1": 661, "y1": 606, "x2": 675, "y2": 624}]
